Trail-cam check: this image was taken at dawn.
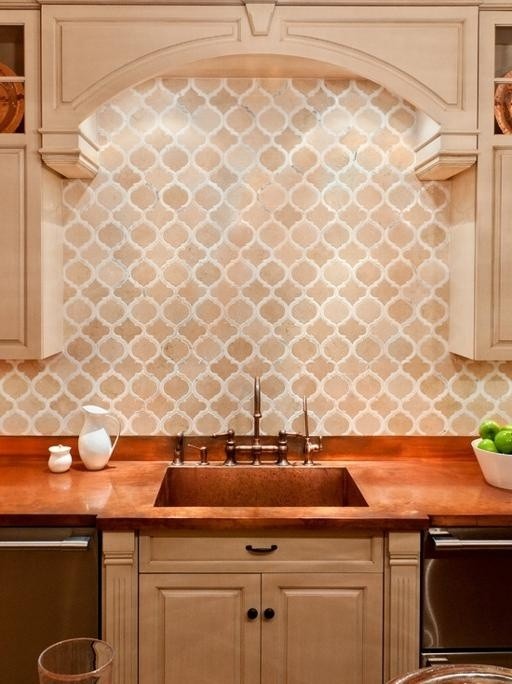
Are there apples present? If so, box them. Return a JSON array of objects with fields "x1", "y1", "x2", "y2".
[{"x1": 477, "y1": 420, "x2": 512, "y2": 454}]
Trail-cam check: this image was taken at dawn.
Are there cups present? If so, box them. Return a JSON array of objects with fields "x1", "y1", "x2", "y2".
[{"x1": 37, "y1": 635, "x2": 119, "y2": 683}]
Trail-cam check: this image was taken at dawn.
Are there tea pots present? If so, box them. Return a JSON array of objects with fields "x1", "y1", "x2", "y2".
[
  {"x1": 81, "y1": 472, "x2": 117, "y2": 510},
  {"x1": 76, "y1": 405, "x2": 122, "y2": 470}
]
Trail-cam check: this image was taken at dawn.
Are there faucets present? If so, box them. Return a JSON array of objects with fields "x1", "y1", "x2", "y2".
[
  {"x1": 251, "y1": 375, "x2": 264, "y2": 464},
  {"x1": 301, "y1": 395, "x2": 313, "y2": 462}
]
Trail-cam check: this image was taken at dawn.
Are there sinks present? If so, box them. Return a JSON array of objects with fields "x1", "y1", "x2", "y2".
[{"x1": 149, "y1": 462, "x2": 372, "y2": 510}]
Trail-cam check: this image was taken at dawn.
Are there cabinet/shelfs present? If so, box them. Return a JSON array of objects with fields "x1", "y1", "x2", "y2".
[
  {"x1": 450, "y1": 1, "x2": 511, "y2": 360},
  {"x1": 101, "y1": 531, "x2": 423, "y2": 684},
  {"x1": 0, "y1": 2, "x2": 64, "y2": 360}
]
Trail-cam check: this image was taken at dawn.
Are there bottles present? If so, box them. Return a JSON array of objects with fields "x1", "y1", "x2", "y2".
[
  {"x1": 50, "y1": 474, "x2": 71, "y2": 491},
  {"x1": 49, "y1": 445, "x2": 72, "y2": 473}
]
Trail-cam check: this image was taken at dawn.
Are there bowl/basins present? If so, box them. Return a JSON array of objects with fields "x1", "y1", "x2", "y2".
[{"x1": 471, "y1": 436, "x2": 510, "y2": 489}]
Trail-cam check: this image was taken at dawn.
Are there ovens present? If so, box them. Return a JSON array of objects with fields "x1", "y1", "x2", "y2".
[{"x1": 422, "y1": 525, "x2": 511, "y2": 670}]
